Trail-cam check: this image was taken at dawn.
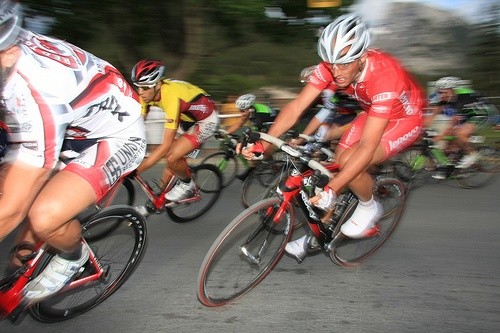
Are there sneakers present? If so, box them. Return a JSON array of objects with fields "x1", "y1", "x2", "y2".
[
  {"x1": 341, "y1": 202, "x2": 378, "y2": 237},
  {"x1": 164, "y1": 179, "x2": 195, "y2": 201},
  {"x1": 431, "y1": 170, "x2": 448, "y2": 180},
  {"x1": 24, "y1": 243, "x2": 89, "y2": 300},
  {"x1": 455, "y1": 153, "x2": 477, "y2": 168},
  {"x1": 285, "y1": 235, "x2": 310, "y2": 263},
  {"x1": 125, "y1": 206, "x2": 150, "y2": 225}
]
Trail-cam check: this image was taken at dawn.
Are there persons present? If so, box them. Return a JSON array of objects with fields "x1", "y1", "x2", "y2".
[
  {"x1": 238, "y1": 11, "x2": 425, "y2": 257},
  {"x1": 422, "y1": 78, "x2": 486, "y2": 180},
  {"x1": 217, "y1": 96, "x2": 281, "y2": 169},
  {"x1": 290, "y1": 63, "x2": 363, "y2": 147},
  {"x1": 132, "y1": 58, "x2": 219, "y2": 202},
  {"x1": 0, "y1": 0, "x2": 145, "y2": 301}
]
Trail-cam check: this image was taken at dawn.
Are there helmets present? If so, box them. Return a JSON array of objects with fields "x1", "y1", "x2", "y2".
[
  {"x1": 131, "y1": 59, "x2": 166, "y2": 88},
  {"x1": 436, "y1": 75, "x2": 459, "y2": 89},
  {"x1": 236, "y1": 93, "x2": 256, "y2": 111},
  {"x1": 317, "y1": 13, "x2": 370, "y2": 64},
  {"x1": 0, "y1": 0, "x2": 24, "y2": 50}
]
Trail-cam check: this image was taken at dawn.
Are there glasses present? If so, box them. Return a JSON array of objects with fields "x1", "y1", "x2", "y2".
[
  {"x1": 438, "y1": 91, "x2": 449, "y2": 95},
  {"x1": 132, "y1": 83, "x2": 157, "y2": 91}
]
